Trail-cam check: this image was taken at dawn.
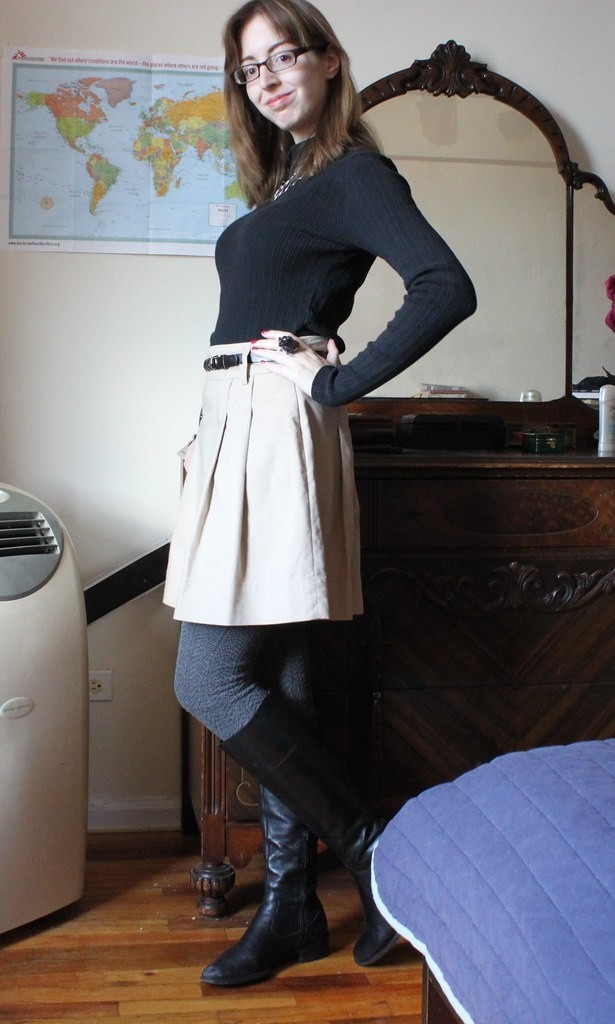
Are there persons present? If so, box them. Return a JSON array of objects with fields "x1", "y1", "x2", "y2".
[{"x1": 162, "y1": 0, "x2": 477, "y2": 986}]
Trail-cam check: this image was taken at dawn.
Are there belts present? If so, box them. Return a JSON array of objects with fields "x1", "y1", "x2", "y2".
[{"x1": 203, "y1": 350, "x2": 329, "y2": 372}]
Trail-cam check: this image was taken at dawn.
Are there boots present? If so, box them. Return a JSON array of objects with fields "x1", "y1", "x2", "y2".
[
  {"x1": 218, "y1": 686, "x2": 400, "y2": 967},
  {"x1": 200, "y1": 715, "x2": 330, "y2": 986}
]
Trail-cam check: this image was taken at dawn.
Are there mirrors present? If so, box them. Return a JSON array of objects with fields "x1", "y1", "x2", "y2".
[{"x1": 335, "y1": 39, "x2": 615, "y2": 408}]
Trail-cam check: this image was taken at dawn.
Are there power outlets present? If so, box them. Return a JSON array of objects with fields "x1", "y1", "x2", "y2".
[{"x1": 88, "y1": 671, "x2": 112, "y2": 701}]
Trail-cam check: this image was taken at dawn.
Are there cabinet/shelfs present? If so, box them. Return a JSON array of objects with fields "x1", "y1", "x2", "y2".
[{"x1": 179, "y1": 395, "x2": 615, "y2": 917}]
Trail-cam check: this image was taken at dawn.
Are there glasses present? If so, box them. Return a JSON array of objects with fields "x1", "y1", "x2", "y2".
[{"x1": 231, "y1": 41, "x2": 329, "y2": 85}]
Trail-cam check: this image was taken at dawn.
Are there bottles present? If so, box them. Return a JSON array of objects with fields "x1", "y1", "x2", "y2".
[{"x1": 598, "y1": 384, "x2": 615, "y2": 458}]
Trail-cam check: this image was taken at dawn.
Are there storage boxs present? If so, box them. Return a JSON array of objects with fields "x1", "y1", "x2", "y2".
[
  {"x1": 419, "y1": 383, "x2": 468, "y2": 398},
  {"x1": 523, "y1": 433, "x2": 560, "y2": 454},
  {"x1": 399, "y1": 414, "x2": 506, "y2": 451}
]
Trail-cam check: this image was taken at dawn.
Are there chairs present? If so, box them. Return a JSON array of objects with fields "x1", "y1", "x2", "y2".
[{"x1": 372, "y1": 739, "x2": 615, "y2": 1024}]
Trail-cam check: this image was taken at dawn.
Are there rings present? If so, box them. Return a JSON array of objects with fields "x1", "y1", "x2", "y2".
[{"x1": 279, "y1": 336, "x2": 301, "y2": 353}]
